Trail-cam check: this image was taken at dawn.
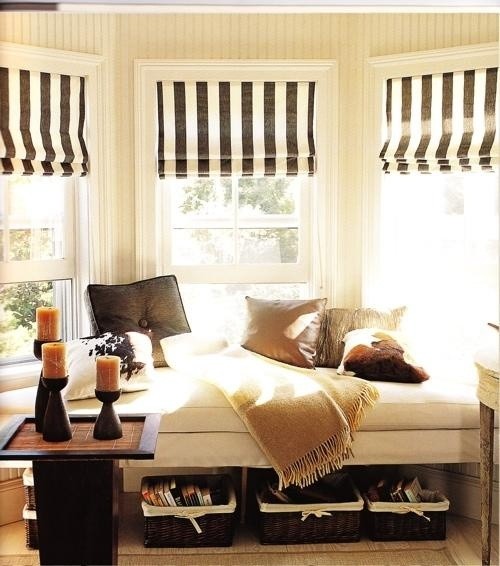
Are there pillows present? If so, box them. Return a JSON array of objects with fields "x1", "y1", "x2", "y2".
[
  {"x1": 314, "y1": 305, "x2": 408, "y2": 368},
  {"x1": 336, "y1": 328, "x2": 430, "y2": 384},
  {"x1": 241, "y1": 296, "x2": 327, "y2": 367},
  {"x1": 84, "y1": 275, "x2": 192, "y2": 367},
  {"x1": 62, "y1": 328, "x2": 154, "y2": 400}
]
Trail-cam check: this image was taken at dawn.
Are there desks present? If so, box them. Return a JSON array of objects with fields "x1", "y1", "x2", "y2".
[{"x1": 472, "y1": 353, "x2": 500, "y2": 566}]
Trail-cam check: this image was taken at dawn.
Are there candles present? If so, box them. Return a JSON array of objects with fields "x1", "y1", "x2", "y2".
[
  {"x1": 96, "y1": 355, "x2": 121, "y2": 392},
  {"x1": 41, "y1": 342, "x2": 68, "y2": 379},
  {"x1": 36, "y1": 307, "x2": 60, "y2": 342}
]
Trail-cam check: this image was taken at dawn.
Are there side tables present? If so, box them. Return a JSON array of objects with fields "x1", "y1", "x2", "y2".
[{"x1": 0, "y1": 413, "x2": 162, "y2": 566}]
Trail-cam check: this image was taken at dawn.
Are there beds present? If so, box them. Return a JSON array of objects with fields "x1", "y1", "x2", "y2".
[{"x1": 0, "y1": 344, "x2": 500, "y2": 525}]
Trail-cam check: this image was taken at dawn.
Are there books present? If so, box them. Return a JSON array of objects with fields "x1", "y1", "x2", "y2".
[
  {"x1": 141, "y1": 476, "x2": 216, "y2": 507},
  {"x1": 365, "y1": 472, "x2": 424, "y2": 502}
]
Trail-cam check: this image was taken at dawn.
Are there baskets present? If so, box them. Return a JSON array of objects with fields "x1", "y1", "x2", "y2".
[
  {"x1": 141, "y1": 473, "x2": 237, "y2": 547},
  {"x1": 22, "y1": 468, "x2": 36, "y2": 507},
  {"x1": 21, "y1": 505, "x2": 39, "y2": 549},
  {"x1": 364, "y1": 479, "x2": 450, "y2": 541},
  {"x1": 256, "y1": 473, "x2": 364, "y2": 544}
]
